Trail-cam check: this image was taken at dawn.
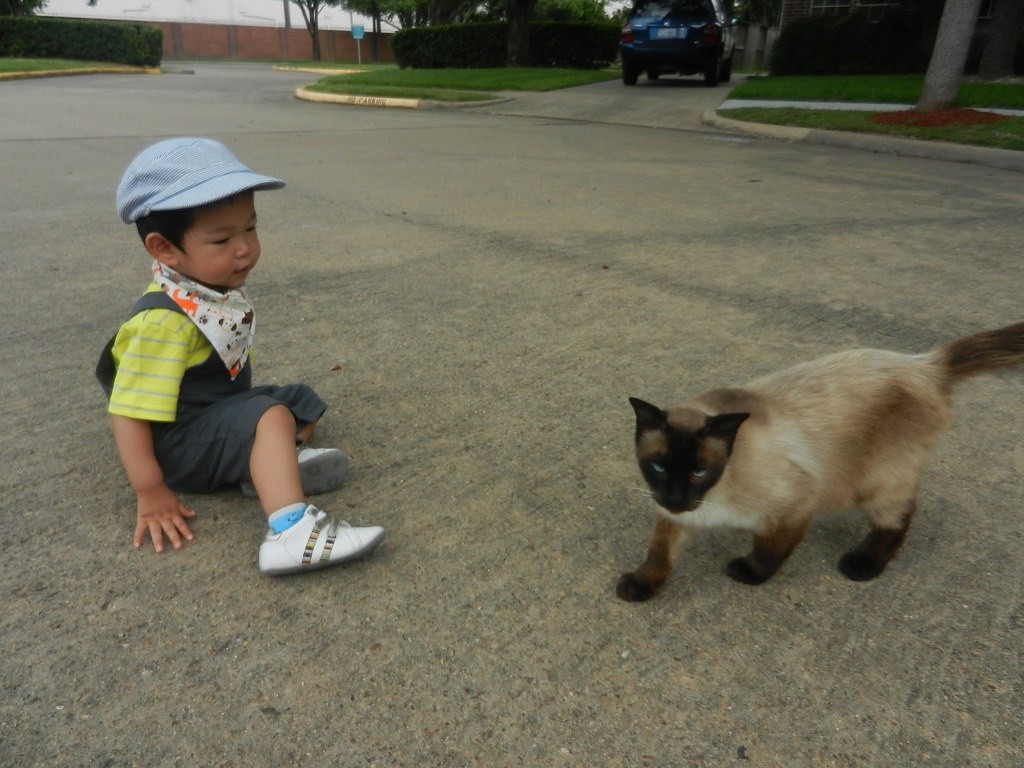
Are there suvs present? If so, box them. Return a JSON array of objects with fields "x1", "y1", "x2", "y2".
[{"x1": 618, "y1": 0, "x2": 739, "y2": 87}]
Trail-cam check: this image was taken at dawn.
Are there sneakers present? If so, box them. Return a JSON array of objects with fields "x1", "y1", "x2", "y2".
[
  {"x1": 258, "y1": 500, "x2": 384, "y2": 574},
  {"x1": 240, "y1": 445, "x2": 348, "y2": 499}
]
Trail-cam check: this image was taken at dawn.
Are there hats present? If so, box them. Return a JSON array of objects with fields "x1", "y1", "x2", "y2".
[{"x1": 117, "y1": 138, "x2": 287, "y2": 224}]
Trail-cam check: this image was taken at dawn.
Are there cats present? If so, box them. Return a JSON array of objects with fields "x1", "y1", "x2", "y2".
[{"x1": 616, "y1": 322, "x2": 1024, "y2": 603}]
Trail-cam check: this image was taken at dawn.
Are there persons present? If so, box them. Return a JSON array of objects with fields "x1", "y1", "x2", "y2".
[{"x1": 94, "y1": 137, "x2": 386, "y2": 576}]
